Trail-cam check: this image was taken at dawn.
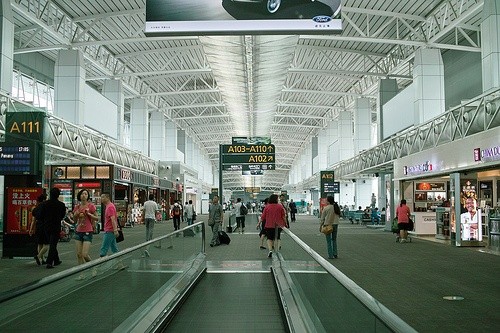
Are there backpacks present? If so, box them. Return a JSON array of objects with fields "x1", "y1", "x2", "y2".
[
  {"x1": 240, "y1": 203, "x2": 247, "y2": 215},
  {"x1": 173, "y1": 204, "x2": 180, "y2": 216}
]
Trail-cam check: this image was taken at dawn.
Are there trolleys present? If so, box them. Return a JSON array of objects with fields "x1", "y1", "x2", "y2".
[{"x1": 391, "y1": 217, "x2": 411, "y2": 243}]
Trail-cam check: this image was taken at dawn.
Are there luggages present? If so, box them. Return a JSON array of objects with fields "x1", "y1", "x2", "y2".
[{"x1": 218, "y1": 231, "x2": 231, "y2": 245}]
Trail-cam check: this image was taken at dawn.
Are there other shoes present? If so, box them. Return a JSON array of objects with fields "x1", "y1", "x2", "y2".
[
  {"x1": 54, "y1": 260, "x2": 62, "y2": 266},
  {"x1": 34, "y1": 255, "x2": 42, "y2": 265},
  {"x1": 334, "y1": 255, "x2": 337, "y2": 258},
  {"x1": 210, "y1": 243, "x2": 214, "y2": 247},
  {"x1": 329, "y1": 256, "x2": 333, "y2": 259},
  {"x1": 268, "y1": 251, "x2": 273, "y2": 257},
  {"x1": 260, "y1": 246, "x2": 266, "y2": 249},
  {"x1": 46, "y1": 265, "x2": 54, "y2": 269},
  {"x1": 42, "y1": 260, "x2": 46, "y2": 264}
]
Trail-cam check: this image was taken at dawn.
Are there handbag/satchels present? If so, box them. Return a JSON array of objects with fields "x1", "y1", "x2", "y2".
[
  {"x1": 321, "y1": 226, "x2": 333, "y2": 233},
  {"x1": 116, "y1": 230, "x2": 124, "y2": 243},
  {"x1": 208, "y1": 219, "x2": 214, "y2": 225}
]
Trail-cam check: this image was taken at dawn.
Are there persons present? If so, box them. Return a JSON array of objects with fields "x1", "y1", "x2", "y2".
[
  {"x1": 396, "y1": 200, "x2": 410, "y2": 244},
  {"x1": 460, "y1": 197, "x2": 479, "y2": 241},
  {"x1": 186, "y1": 200, "x2": 195, "y2": 225},
  {"x1": 72, "y1": 189, "x2": 98, "y2": 265},
  {"x1": 319, "y1": 196, "x2": 339, "y2": 259},
  {"x1": 208, "y1": 194, "x2": 223, "y2": 247},
  {"x1": 100, "y1": 192, "x2": 120, "y2": 257},
  {"x1": 29, "y1": 194, "x2": 48, "y2": 265},
  {"x1": 143, "y1": 194, "x2": 158, "y2": 241},
  {"x1": 32, "y1": 188, "x2": 66, "y2": 267},
  {"x1": 183, "y1": 202, "x2": 188, "y2": 222},
  {"x1": 343, "y1": 204, "x2": 385, "y2": 225},
  {"x1": 171, "y1": 199, "x2": 182, "y2": 230},
  {"x1": 234, "y1": 198, "x2": 245, "y2": 233},
  {"x1": 222, "y1": 193, "x2": 296, "y2": 257}
]
